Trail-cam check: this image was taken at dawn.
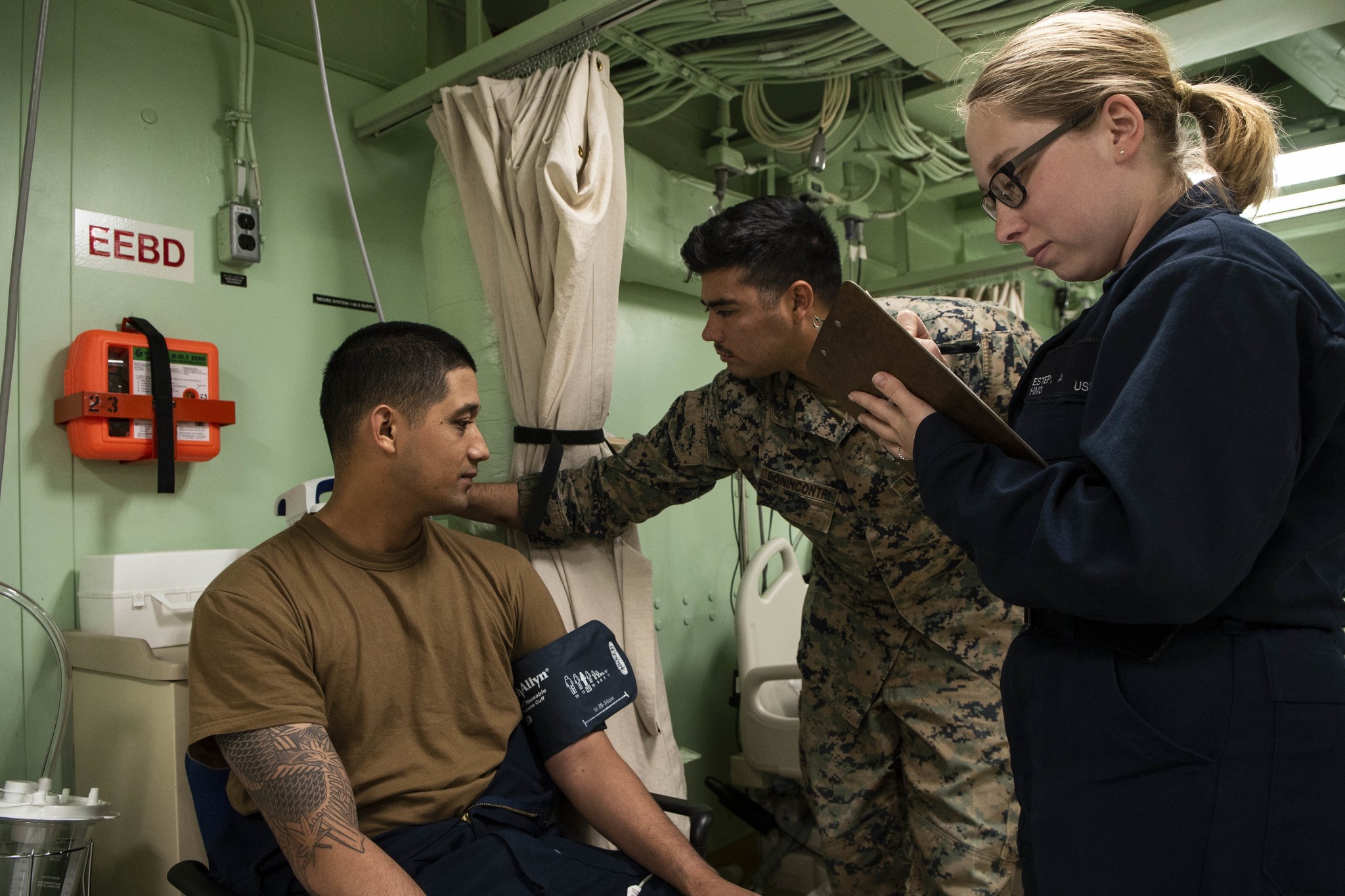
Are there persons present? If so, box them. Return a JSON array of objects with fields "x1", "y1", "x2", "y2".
[
  {"x1": 441, "y1": 193, "x2": 1041, "y2": 896},
  {"x1": 190, "y1": 321, "x2": 760, "y2": 896},
  {"x1": 848, "y1": 12, "x2": 1345, "y2": 896}
]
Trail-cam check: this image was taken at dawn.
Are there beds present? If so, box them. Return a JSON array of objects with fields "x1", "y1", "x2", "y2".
[{"x1": 735, "y1": 536, "x2": 814, "y2": 891}]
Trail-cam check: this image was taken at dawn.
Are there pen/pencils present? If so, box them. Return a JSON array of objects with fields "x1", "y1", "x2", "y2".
[{"x1": 937, "y1": 339, "x2": 979, "y2": 356}]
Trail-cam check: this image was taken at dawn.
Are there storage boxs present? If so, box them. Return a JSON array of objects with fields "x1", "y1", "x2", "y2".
[{"x1": 77, "y1": 546, "x2": 249, "y2": 649}]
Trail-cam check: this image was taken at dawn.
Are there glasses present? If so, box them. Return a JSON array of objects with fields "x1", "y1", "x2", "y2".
[{"x1": 981, "y1": 111, "x2": 1090, "y2": 222}]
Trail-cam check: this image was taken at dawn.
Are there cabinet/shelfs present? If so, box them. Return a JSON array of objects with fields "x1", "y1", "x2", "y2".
[{"x1": 64, "y1": 629, "x2": 208, "y2": 896}]
